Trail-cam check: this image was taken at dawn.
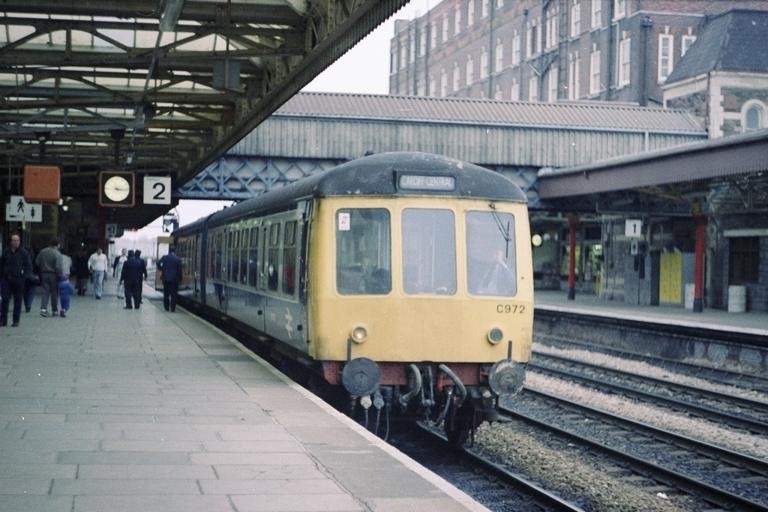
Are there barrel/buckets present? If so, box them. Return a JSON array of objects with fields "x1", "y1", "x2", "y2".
[{"x1": 728, "y1": 285, "x2": 746, "y2": 312}]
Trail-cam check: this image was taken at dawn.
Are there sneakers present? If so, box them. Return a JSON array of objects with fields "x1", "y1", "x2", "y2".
[
  {"x1": 96, "y1": 296, "x2": 101, "y2": 300},
  {"x1": 40, "y1": 310, "x2": 65, "y2": 317},
  {"x1": 26, "y1": 308, "x2": 30, "y2": 313},
  {"x1": 11, "y1": 322, "x2": 19, "y2": 327}
]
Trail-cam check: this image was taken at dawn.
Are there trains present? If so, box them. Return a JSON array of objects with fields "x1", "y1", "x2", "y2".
[{"x1": 153, "y1": 149, "x2": 534, "y2": 450}]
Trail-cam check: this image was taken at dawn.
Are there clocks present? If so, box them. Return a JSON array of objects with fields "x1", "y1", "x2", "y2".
[{"x1": 99, "y1": 171, "x2": 136, "y2": 208}]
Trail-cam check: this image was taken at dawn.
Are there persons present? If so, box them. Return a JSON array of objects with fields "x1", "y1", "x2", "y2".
[
  {"x1": 116, "y1": 249, "x2": 143, "y2": 309},
  {"x1": 35, "y1": 240, "x2": 65, "y2": 317},
  {"x1": 22, "y1": 247, "x2": 35, "y2": 312},
  {"x1": 112, "y1": 248, "x2": 129, "y2": 301},
  {"x1": 88, "y1": 247, "x2": 108, "y2": 299},
  {"x1": 156, "y1": 244, "x2": 183, "y2": 312},
  {"x1": 75, "y1": 248, "x2": 90, "y2": 297},
  {"x1": 134, "y1": 249, "x2": 148, "y2": 303},
  {"x1": 0, "y1": 230, "x2": 33, "y2": 327},
  {"x1": 57, "y1": 247, "x2": 75, "y2": 317}
]
